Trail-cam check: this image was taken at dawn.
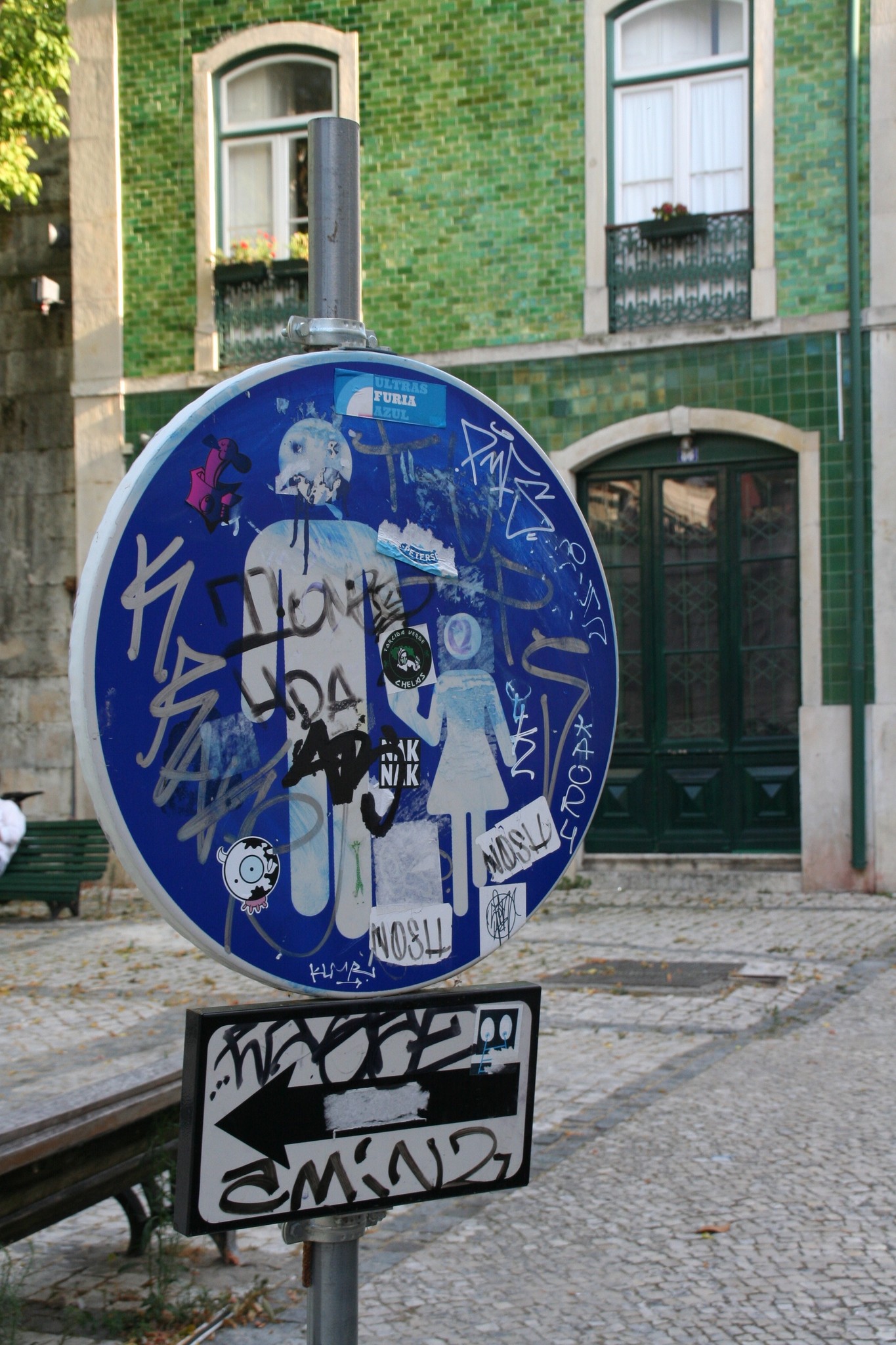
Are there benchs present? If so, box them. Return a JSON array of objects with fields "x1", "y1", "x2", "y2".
[
  {"x1": 0, "y1": 1054, "x2": 248, "y2": 1268},
  {"x1": 0, "y1": 816, "x2": 112, "y2": 920}
]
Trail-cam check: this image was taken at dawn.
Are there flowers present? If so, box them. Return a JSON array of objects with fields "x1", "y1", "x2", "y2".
[
  {"x1": 289, "y1": 231, "x2": 310, "y2": 263},
  {"x1": 651, "y1": 202, "x2": 693, "y2": 221},
  {"x1": 210, "y1": 229, "x2": 274, "y2": 268}
]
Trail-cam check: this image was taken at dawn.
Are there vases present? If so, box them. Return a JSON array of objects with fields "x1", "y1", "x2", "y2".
[
  {"x1": 271, "y1": 256, "x2": 310, "y2": 279},
  {"x1": 212, "y1": 261, "x2": 267, "y2": 287},
  {"x1": 636, "y1": 213, "x2": 709, "y2": 239}
]
